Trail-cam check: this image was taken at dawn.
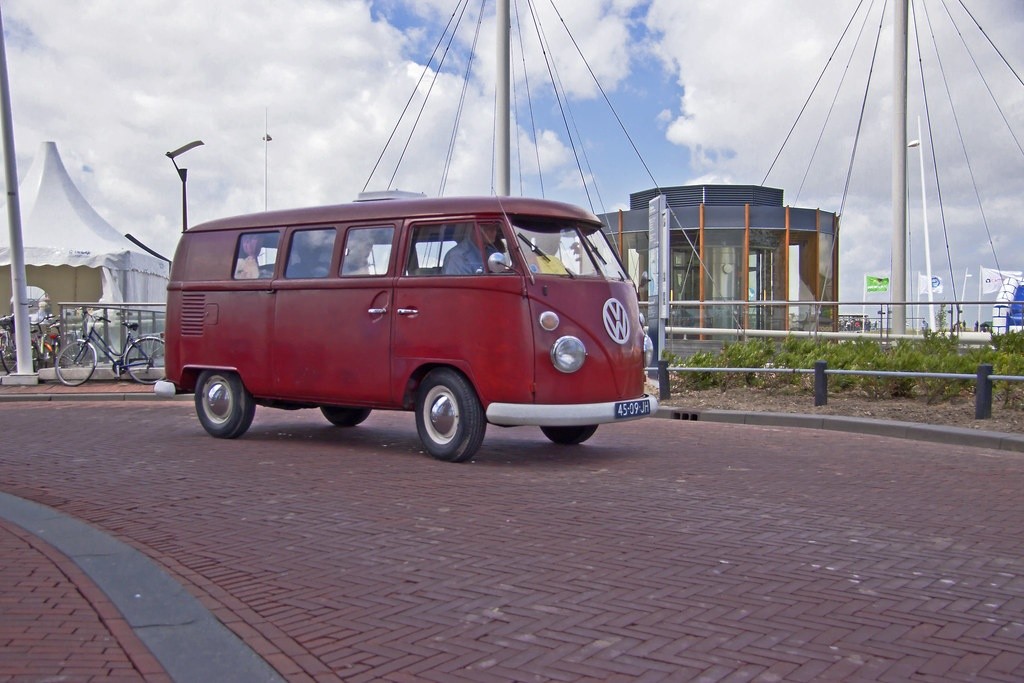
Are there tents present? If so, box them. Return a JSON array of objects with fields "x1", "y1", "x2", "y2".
[{"x1": 0, "y1": 141, "x2": 172, "y2": 368}]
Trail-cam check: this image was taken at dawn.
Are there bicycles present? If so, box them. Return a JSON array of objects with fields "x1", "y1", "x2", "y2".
[
  {"x1": 0, "y1": 304, "x2": 81, "y2": 373},
  {"x1": 55, "y1": 310, "x2": 167, "y2": 386}
]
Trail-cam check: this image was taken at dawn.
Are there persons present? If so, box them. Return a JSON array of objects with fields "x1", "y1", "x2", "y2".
[
  {"x1": 527, "y1": 232, "x2": 561, "y2": 273},
  {"x1": 234, "y1": 233, "x2": 262, "y2": 280},
  {"x1": 441, "y1": 223, "x2": 503, "y2": 276},
  {"x1": 922, "y1": 321, "x2": 928, "y2": 331},
  {"x1": 837, "y1": 319, "x2": 877, "y2": 332},
  {"x1": 962, "y1": 320, "x2": 966, "y2": 331},
  {"x1": 975, "y1": 321, "x2": 979, "y2": 333},
  {"x1": 343, "y1": 235, "x2": 374, "y2": 275}
]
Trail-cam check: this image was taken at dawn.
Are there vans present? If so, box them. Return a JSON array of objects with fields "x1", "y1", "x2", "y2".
[{"x1": 152, "y1": 188, "x2": 659, "y2": 462}]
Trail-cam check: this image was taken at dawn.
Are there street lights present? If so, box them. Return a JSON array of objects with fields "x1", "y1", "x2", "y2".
[{"x1": 909, "y1": 114, "x2": 936, "y2": 331}]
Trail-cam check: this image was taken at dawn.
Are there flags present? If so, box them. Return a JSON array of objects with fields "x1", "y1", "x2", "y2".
[
  {"x1": 980, "y1": 268, "x2": 1022, "y2": 295},
  {"x1": 864, "y1": 276, "x2": 889, "y2": 292},
  {"x1": 918, "y1": 274, "x2": 942, "y2": 294}
]
